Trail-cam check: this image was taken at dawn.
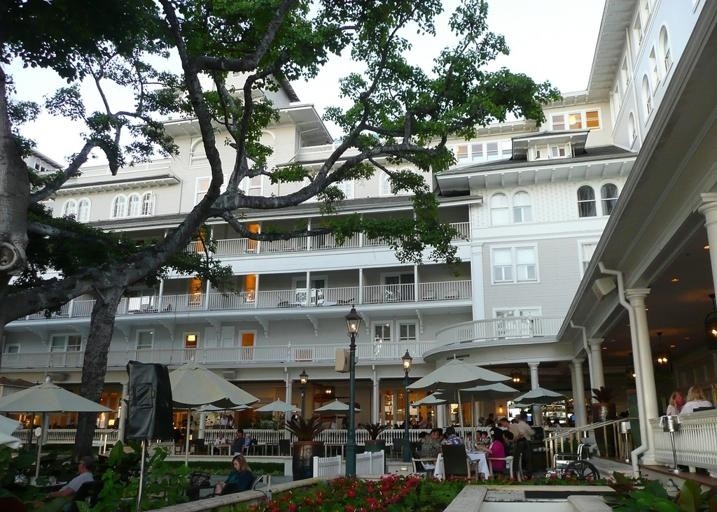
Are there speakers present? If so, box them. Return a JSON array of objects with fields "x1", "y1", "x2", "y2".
[
  {"x1": 223, "y1": 371, "x2": 235, "y2": 379},
  {"x1": 55, "y1": 373, "x2": 65, "y2": 381},
  {"x1": 591, "y1": 277, "x2": 616, "y2": 300}
]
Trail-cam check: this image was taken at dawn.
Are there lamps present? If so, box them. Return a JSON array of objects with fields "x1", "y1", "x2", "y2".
[
  {"x1": 705, "y1": 293, "x2": 717, "y2": 336},
  {"x1": 654, "y1": 332, "x2": 669, "y2": 367},
  {"x1": 511, "y1": 363, "x2": 522, "y2": 382}
]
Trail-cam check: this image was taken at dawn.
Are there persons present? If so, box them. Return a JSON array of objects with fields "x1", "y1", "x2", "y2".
[
  {"x1": 680, "y1": 385, "x2": 713, "y2": 413},
  {"x1": 666, "y1": 390, "x2": 684, "y2": 414},
  {"x1": 173, "y1": 412, "x2": 251, "y2": 454},
  {"x1": 214, "y1": 456, "x2": 253, "y2": 496},
  {"x1": 380, "y1": 408, "x2": 578, "y2": 482},
  {"x1": 32, "y1": 454, "x2": 97, "y2": 511}
]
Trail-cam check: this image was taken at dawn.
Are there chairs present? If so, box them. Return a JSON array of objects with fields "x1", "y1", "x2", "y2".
[
  {"x1": 423, "y1": 289, "x2": 434, "y2": 299},
  {"x1": 134, "y1": 304, "x2": 170, "y2": 312},
  {"x1": 445, "y1": 290, "x2": 459, "y2": 298},
  {"x1": 694, "y1": 406, "x2": 714, "y2": 412}
]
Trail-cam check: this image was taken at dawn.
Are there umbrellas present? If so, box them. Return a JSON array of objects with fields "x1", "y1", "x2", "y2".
[
  {"x1": 313, "y1": 398, "x2": 361, "y2": 430},
  {"x1": 412, "y1": 354, "x2": 567, "y2": 441},
  {"x1": 166, "y1": 359, "x2": 260, "y2": 472},
  {"x1": 0, "y1": 375, "x2": 118, "y2": 480},
  {"x1": 255, "y1": 397, "x2": 301, "y2": 441}
]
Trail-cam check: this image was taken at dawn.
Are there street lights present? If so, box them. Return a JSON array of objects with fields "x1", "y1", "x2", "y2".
[
  {"x1": 298, "y1": 368, "x2": 310, "y2": 422},
  {"x1": 399, "y1": 347, "x2": 417, "y2": 463},
  {"x1": 343, "y1": 300, "x2": 366, "y2": 480}
]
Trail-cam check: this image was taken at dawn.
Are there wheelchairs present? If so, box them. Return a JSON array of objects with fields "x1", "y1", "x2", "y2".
[{"x1": 548, "y1": 440, "x2": 602, "y2": 480}]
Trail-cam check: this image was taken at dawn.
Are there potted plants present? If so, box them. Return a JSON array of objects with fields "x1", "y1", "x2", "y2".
[
  {"x1": 282, "y1": 415, "x2": 325, "y2": 481},
  {"x1": 363, "y1": 424, "x2": 391, "y2": 475},
  {"x1": 591, "y1": 387, "x2": 616, "y2": 456}
]
